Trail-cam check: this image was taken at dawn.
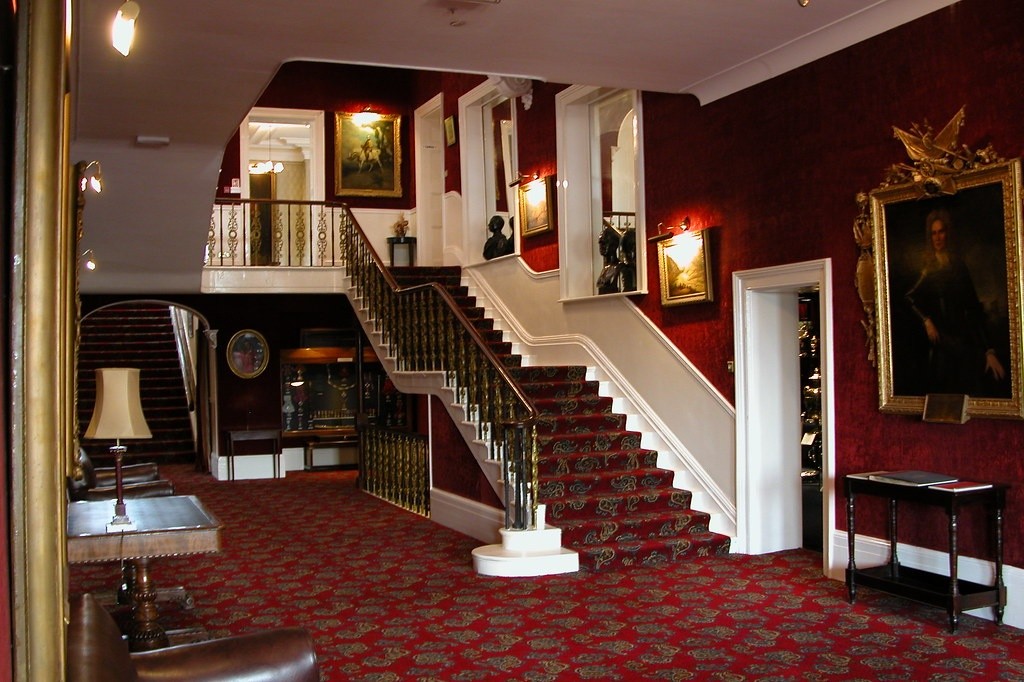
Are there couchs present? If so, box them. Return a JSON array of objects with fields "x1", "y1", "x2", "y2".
[{"x1": 65, "y1": 592, "x2": 322, "y2": 682}]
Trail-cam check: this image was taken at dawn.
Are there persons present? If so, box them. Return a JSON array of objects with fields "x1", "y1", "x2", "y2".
[
  {"x1": 620, "y1": 231, "x2": 636, "y2": 292},
  {"x1": 597, "y1": 228, "x2": 625, "y2": 294},
  {"x1": 508, "y1": 216, "x2": 514, "y2": 255},
  {"x1": 483, "y1": 216, "x2": 508, "y2": 260}
]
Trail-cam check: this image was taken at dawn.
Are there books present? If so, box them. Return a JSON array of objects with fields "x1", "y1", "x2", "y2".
[{"x1": 846, "y1": 470, "x2": 993, "y2": 493}]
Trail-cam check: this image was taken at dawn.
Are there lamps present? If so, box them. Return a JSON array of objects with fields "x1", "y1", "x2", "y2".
[
  {"x1": 284, "y1": 368, "x2": 305, "y2": 387},
  {"x1": 508, "y1": 170, "x2": 540, "y2": 190},
  {"x1": 85, "y1": 367, "x2": 154, "y2": 532},
  {"x1": 248, "y1": 158, "x2": 284, "y2": 175},
  {"x1": 111, "y1": 1, "x2": 140, "y2": 56},
  {"x1": 136, "y1": 136, "x2": 171, "y2": 148},
  {"x1": 648, "y1": 216, "x2": 691, "y2": 242},
  {"x1": 80, "y1": 157, "x2": 103, "y2": 195},
  {"x1": 81, "y1": 249, "x2": 96, "y2": 272}
]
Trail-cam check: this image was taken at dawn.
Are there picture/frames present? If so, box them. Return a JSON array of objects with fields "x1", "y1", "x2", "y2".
[
  {"x1": 226, "y1": 330, "x2": 270, "y2": 380},
  {"x1": 332, "y1": 110, "x2": 403, "y2": 198},
  {"x1": 63, "y1": 90, "x2": 85, "y2": 484},
  {"x1": 656, "y1": 228, "x2": 714, "y2": 310},
  {"x1": 443, "y1": 111, "x2": 458, "y2": 149},
  {"x1": 518, "y1": 175, "x2": 554, "y2": 237},
  {"x1": 853, "y1": 104, "x2": 1024, "y2": 422}
]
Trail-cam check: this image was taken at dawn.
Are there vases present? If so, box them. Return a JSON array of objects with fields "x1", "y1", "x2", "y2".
[{"x1": 396, "y1": 227, "x2": 405, "y2": 236}]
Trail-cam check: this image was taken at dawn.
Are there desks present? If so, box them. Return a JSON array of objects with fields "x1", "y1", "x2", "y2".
[
  {"x1": 844, "y1": 469, "x2": 1012, "y2": 637},
  {"x1": 67, "y1": 493, "x2": 230, "y2": 653},
  {"x1": 220, "y1": 419, "x2": 285, "y2": 485},
  {"x1": 386, "y1": 237, "x2": 417, "y2": 267}
]
